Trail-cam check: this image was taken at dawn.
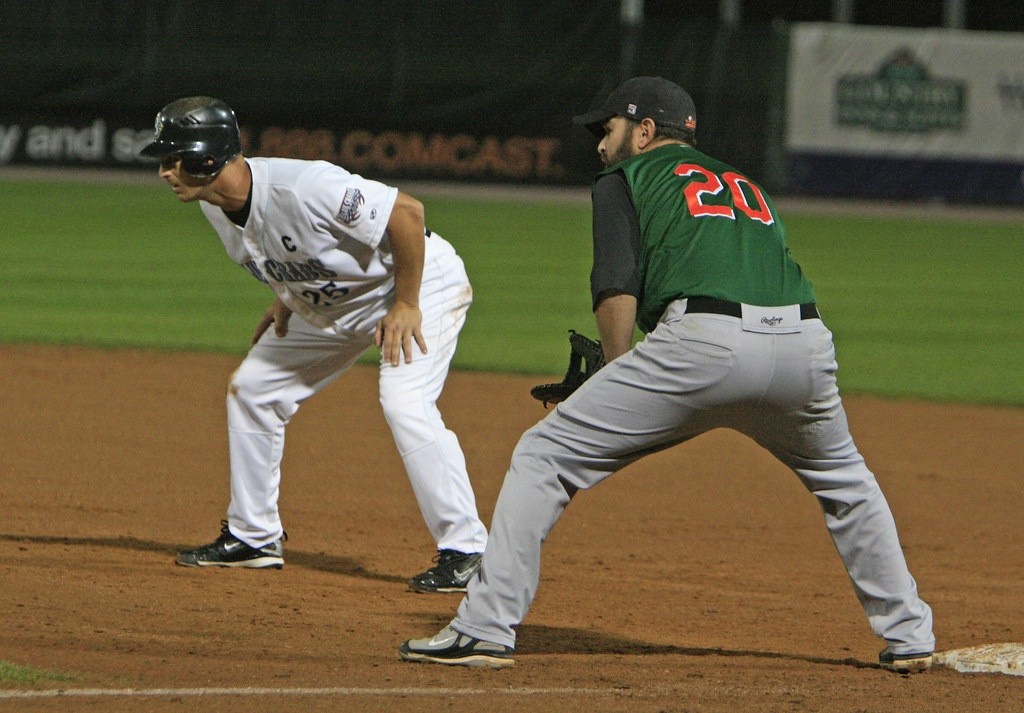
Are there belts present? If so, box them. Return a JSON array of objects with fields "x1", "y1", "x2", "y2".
[
  {"x1": 685, "y1": 295, "x2": 819, "y2": 320},
  {"x1": 426, "y1": 228, "x2": 432, "y2": 237}
]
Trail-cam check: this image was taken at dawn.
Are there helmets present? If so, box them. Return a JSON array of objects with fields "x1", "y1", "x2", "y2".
[{"x1": 139, "y1": 96, "x2": 242, "y2": 188}]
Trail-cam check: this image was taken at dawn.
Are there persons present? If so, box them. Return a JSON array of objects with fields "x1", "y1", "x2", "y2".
[
  {"x1": 139, "y1": 97, "x2": 489, "y2": 592},
  {"x1": 399, "y1": 77, "x2": 936, "y2": 672}
]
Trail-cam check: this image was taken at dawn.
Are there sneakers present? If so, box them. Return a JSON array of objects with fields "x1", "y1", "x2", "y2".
[
  {"x1": 409, "y1": 549, "x2": 483, "y2": 593},
  {"x1": 878, "y1": 645, "x2": 933, "y2": 672},
  {"x1": 176, "y1": 520, "x2": 288, "y2": 569},
  {"x1": 399, "y1": 624, "x2": 514, "y2": 669}
]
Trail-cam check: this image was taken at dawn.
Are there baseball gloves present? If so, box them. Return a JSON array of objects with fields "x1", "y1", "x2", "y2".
[{"x1": 530, "y1": 328, "x2": 608, "y2": 405}]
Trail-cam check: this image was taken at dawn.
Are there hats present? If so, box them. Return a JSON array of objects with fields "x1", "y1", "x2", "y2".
[{"x1": 571, "y1": 76, "x2": 697, "y2": 134}]
